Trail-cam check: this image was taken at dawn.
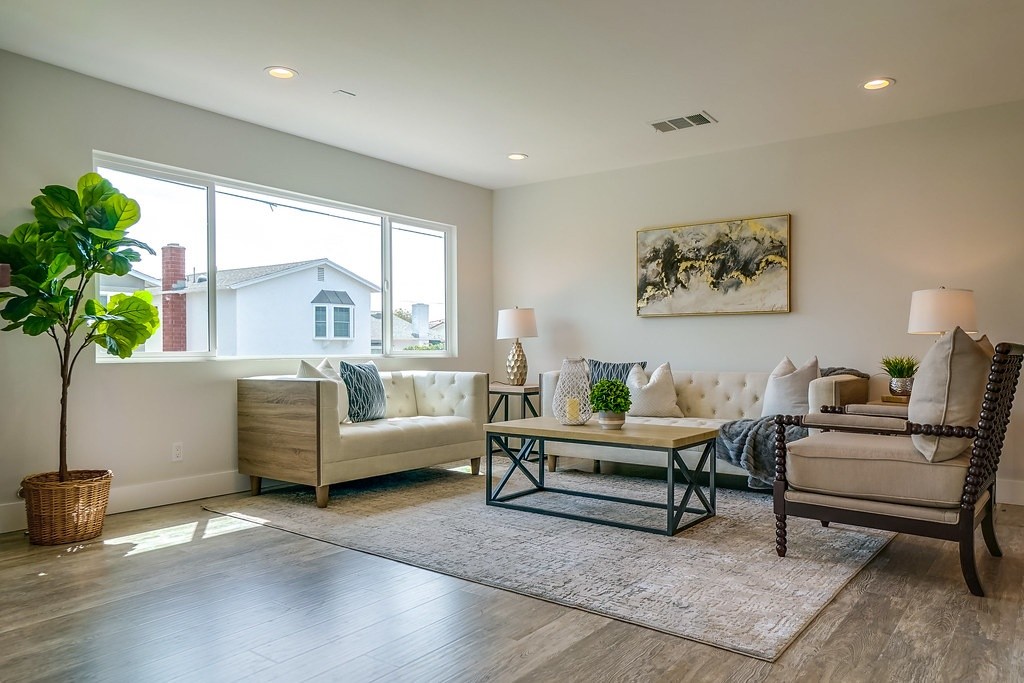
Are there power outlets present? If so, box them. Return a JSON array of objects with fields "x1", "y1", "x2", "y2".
[{"x1": 172, "y1": 442, "x2": 183, "y2": 461}]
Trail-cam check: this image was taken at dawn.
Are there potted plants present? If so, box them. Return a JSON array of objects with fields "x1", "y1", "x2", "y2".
[
  {"x1": 589, "y1": 378, "x2": 632, "y2": 429},
  {"x1": 0, "y1": 171, "x2": 160, "y2": 544},
  {"x1": 875, "y1": 354, "x2": 920, "y2": 396}
]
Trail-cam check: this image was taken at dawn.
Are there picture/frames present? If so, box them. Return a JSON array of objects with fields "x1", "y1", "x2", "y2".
[{"x1": 636, "y1": 213, "x2": 791, "y2": 317}]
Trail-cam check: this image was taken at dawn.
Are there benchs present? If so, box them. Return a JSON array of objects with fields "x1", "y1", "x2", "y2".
[
  {"x1": 237, "y1": 370, "x2": 489, "y2": 507},
  {"x1": 541, "y1": 369, "x2": 868, "y2": 494}
]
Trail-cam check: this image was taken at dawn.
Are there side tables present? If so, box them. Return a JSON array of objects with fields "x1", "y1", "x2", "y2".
[
  {"x1": 881, "y1": 394, "x2": 909, "y2": 404},
  {"x1": 490, "y1": 384, "x2": 546, "y2": 462}
]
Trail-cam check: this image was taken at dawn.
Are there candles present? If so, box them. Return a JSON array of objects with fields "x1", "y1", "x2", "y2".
[{"x1": 567, "y1": 396, "x2": 579, "y2": 421}]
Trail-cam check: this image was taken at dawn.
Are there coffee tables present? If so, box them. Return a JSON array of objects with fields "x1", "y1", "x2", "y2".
[{"x1": 483, "y1": 417, "x2": 719, "y2": 535}]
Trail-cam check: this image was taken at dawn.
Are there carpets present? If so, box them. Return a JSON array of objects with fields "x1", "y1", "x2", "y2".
[{"x1": 202, "y1": 453, "x2": 898, "y2": 662}]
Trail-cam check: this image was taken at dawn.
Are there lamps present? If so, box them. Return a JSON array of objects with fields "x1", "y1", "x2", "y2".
[
  {"x1": 497, "y1": 305, "x2": 539, "y2": 386},
  {"x1": 907, "y1": 284, "x2": 978, "y2": 337}
]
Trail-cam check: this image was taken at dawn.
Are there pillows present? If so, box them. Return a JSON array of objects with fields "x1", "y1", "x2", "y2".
[
  {"x1": 296, "y1": 360, "x2": 331, "y2": 379},
  {"x1": 316, "y1": 357, "x2": 350, "y2": 423},
  {"x1": 338, "y1": 359, "x2": 387, "y2": 422},
  {"x1": 587, "y1": 359, "x2": 647, "y2": 384},
  {"x1": 625, "y1": 362, "x2": 685, "y2": 418},
  {"x1": 761, "y1": 355, "x2": 821, "y2": 415}
]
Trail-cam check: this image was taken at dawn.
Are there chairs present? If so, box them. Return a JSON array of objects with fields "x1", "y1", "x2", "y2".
[
  {"x1": 908, "y1": 326, "x2": 995, "y2": 463},
  {"x1": 773, "y1": 341, "x2": 1024, "y2": 596}
]
